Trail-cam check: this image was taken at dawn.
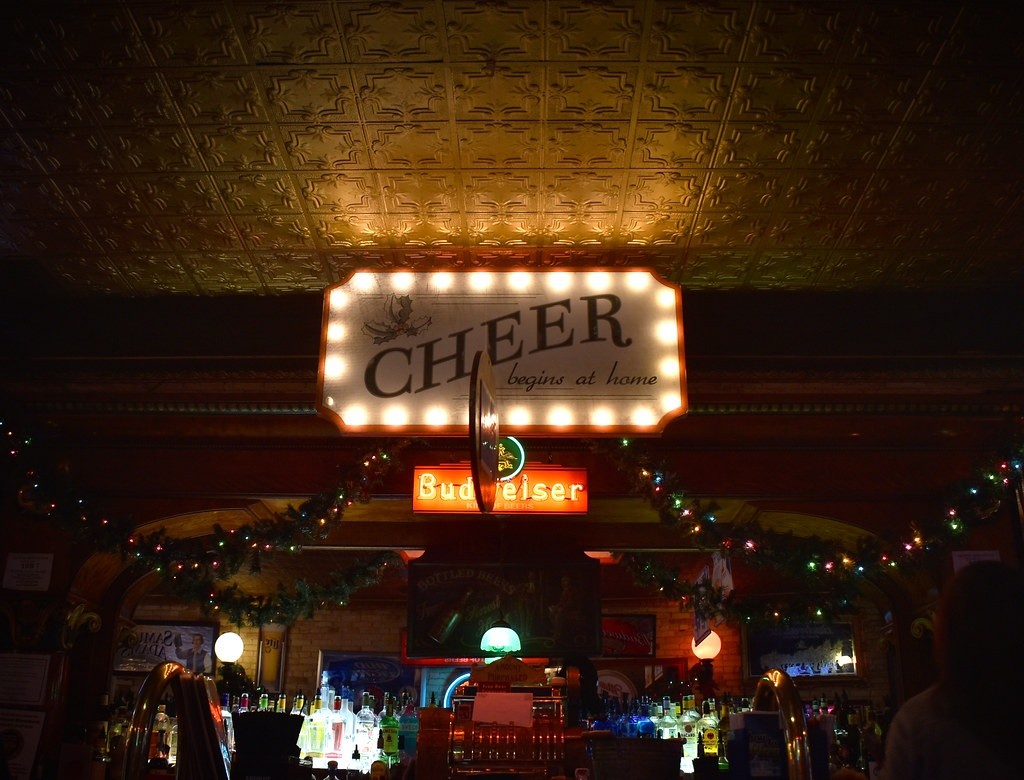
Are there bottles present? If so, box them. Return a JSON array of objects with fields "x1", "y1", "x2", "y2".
[
  {"x1": 427, "y1": 692, "x2": 438, "y2": 708},
  {"x1": 89, "y1": 692, "x2": 129, "y2": 762},
  {"x1": 148, "y1": 705, "x2": 179, "y2": 765},
  {"x1": 425, "y1": 589, "x2": 470, "y2": 645},
  {"x1": 574, "y1": 767, "x2": 590, "y2": 780},
  {"x1": 804, "y1": 689, "x2": 883, "y2": 771},
  {"x1": 589, "y1": 690, "x2": 751, "y2": 774},
  {"x1": 218, "y1": 686, "x2": 421, "y2": 780}
]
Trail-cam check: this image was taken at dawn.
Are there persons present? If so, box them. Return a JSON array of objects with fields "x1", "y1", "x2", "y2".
[{"x1": 876, "y1": 559, "x2": 1024, "y2": 780}]
